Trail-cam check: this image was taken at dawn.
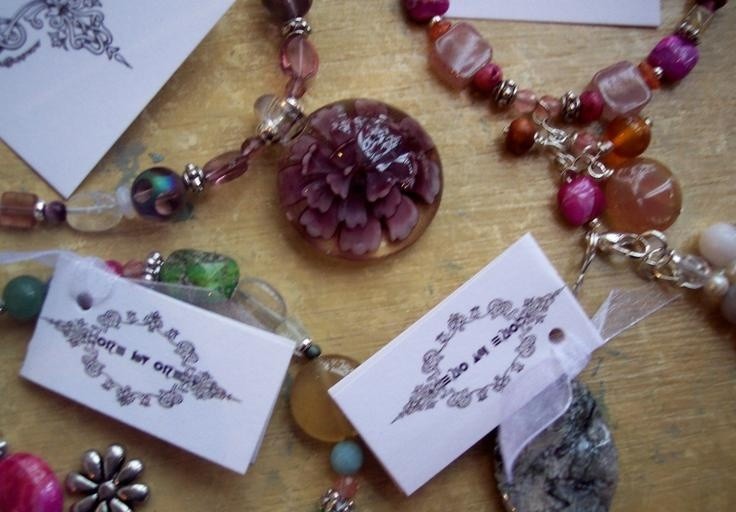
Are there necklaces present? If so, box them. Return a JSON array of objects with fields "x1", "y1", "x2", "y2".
[{"x1": 2, "y1": 2, "x2": 735, "y2": 512}]
